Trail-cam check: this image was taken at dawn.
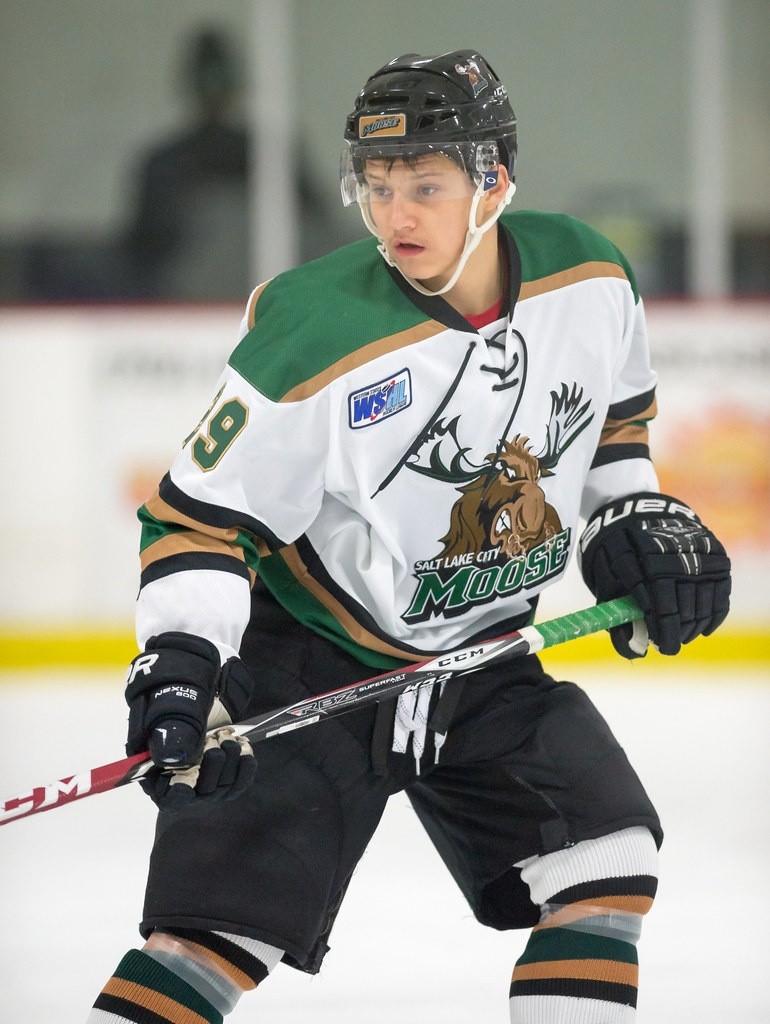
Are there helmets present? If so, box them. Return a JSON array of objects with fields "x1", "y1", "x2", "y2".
[{"x1": 343, "y1": 49, "x2": 519, "y2": 185}]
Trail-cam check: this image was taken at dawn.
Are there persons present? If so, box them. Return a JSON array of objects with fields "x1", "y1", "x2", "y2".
[
  {"x1": 124, "y1": 29, "x2": 330, "y2": 301},
  {"x1": 90, "y1": 48, "x2": 732, "y2": 1024}
]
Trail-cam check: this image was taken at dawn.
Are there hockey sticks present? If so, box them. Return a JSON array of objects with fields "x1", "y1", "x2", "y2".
[{"x1": 1, "y1": 595, "x2": 645, "y2": 830}]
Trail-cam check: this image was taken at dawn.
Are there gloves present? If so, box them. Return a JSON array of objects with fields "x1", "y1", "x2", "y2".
[
  {"x1": 574, "y1": 491, "x2": 733, "y2": 660},
  {"x1": 122, "y1": 629, "x2": 259, "y2": 815}
]
